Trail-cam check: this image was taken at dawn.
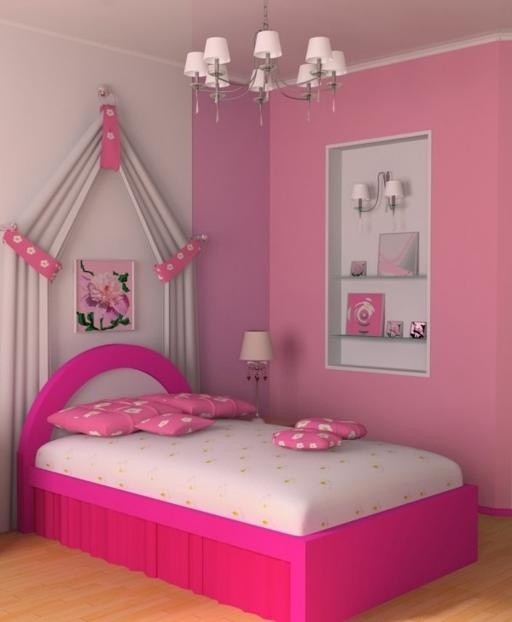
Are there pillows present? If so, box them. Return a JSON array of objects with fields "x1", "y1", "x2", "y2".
[
  {"x1": 295, "y1": 416, "x2": 366, "y2": 441},
  {"x1": 140, "y1": 392, "x2": 259, "y2": 420},
  {"x1": 270, "y1": 426, "x2": 344, "y2": 451},
  {"x1": 133, "y1": 411, "x2": 218, "y2": 439},
  {"x1": 46, "y1": 396, "x2": 184, "y2": 439}
]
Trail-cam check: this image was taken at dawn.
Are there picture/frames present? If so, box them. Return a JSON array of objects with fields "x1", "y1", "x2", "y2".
[{"x1": 344, "y1": 232, "x2": 426, "y2": 337}]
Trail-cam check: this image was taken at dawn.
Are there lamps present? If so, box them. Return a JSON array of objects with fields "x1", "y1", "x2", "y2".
[
  {"x1": 183, "y1": 0, "x2": 348, "y2": 128},
  {"x1": 349, "y1": 169, "x2": 405, "y2": 218},
  {"x1": 239, "y1": 330, "x2": 274, "y2": 425}
]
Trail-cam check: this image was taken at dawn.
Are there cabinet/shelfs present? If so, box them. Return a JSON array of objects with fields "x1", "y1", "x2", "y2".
[{"x1": 324, "y1": 130, "x2": 432, "y2": 376}]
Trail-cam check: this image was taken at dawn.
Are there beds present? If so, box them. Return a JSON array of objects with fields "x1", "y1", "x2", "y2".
[{"x1": 15, "y1": 344, "x2": 478, "y2": 620}]
